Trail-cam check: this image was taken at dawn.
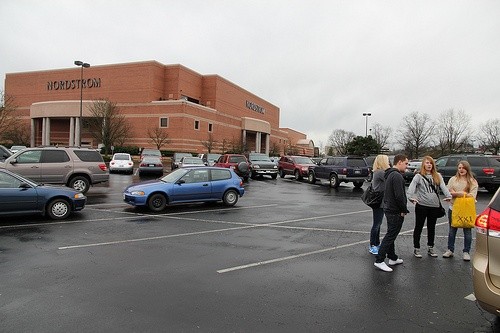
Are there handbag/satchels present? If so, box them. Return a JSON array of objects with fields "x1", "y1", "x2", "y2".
[
  {"x1": 437, "y1": 207, "x2": 445, "y2": 218},
  {"x1": 451, "y1": 198, "x2": 476, "y2": 227},
  {"x1": 361, "y1": 187, "x2": 384, "y2": 208}
]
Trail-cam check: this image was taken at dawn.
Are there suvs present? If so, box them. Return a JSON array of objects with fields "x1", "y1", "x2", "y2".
[
  {"x1": 197, "y1": 153, "x2": 252, "y2": 181},
  {"x1": 471, "y1": 187, "x2": 500, "y2": 316},
  {"x1": 0, "y1": 145, "x2": 110, "y2": 193},
  {"x1": 271, "y1": 156, "x2": 371, "y2": 188},
  {"x1": 171, "y1": 152, "x2": 193, "y2": 170},
  {"x1": 245, "y1": 153, "x2": 280, "y2": 179}
]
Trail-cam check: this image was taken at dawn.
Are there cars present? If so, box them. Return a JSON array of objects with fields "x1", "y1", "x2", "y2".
[
  {"x1": 178, "y1": 156, "x2": 206, "y2": 167},
  {"x1": 138, "y1": 149, "x2": 166, "y2": 176},
  {"x1": 109, "y1": 153, "x2": 135, "y2": 174},
  {"x1": 366, "y1": 155, "x2": 500, "y2": 192},
  {"x1": 122, "y1": 167, "x2": 246, "y2": 212},
  {"x1": 0, "y1": 167, "x2": 87, "y2": 220}
]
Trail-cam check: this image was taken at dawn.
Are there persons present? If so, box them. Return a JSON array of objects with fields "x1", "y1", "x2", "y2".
[
  {"x1": 406, "y1": 156, "x2": 452, "y2": 258},
  {"x1": 373, "y1": 154, "x2": 410, "y2": 271},
  {"x1": 440, "y1": 160, "x2": 478, "y2": 261},
  {"x1": 369, "y1": 154, "x2": 390, "y2": 254}
]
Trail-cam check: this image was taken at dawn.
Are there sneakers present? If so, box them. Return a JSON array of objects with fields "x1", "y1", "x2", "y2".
[
  {"x1": 369, "y1": 243, "x2": 381, "y2": 254},
  {"x1": 414, "y1": 247, "x2": 423, "y2": 257},
  {"x1": 463, "y1": 252, "x2": 471, "y2": 260},
  {"x1": 427, "y1": 246, "x2": 438, "y2": 256},
  {"x1": 443, "y1": 249, "x2": 454, "y2": 257},
  {"x1": 388, "y1": 257, "x2": 403, "y2": 265},
  {"x1": 373, "y1": 261, "x2": 393, "y2": 271}
]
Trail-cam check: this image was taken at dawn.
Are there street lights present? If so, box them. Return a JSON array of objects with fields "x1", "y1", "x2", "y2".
[
  {"x1": 74, "y1": 60, "x2": 90, "y2": 148},
  {"x1": 363, "y1": 113, "x2": 372, "y2": 137}
]
